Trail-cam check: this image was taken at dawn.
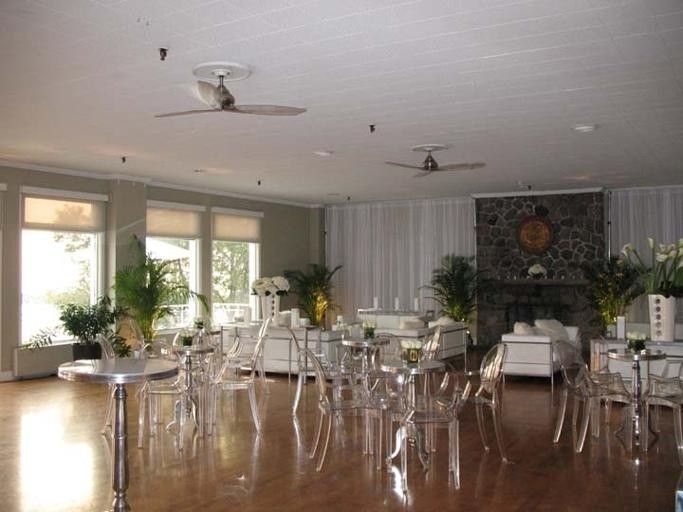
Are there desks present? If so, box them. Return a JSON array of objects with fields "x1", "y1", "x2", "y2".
[{"x1": 58, "y1": 358, "x2": 179, "y2": 512}]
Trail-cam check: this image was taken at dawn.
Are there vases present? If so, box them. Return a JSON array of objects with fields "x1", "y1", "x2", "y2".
[
  {"x1": 648, "y1": 294, "x2": 678, "y2": 342},
  {"x1": 533, "y1": 274, "x2": 542, "y2": 280},
  {"x1": 261, "y1": 296, "x2": 280, "y2": 326}
]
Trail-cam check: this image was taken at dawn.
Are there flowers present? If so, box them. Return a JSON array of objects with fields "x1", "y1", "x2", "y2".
[
  {"x1": 528, "y1": 263, "x2": 546, "y2": 277},
  {"x1": 251, "y1": 277, "x2": 290, "y2": 297},
  {"x1": 621, "y1": 237, "x2": 683, "y2": 298}
]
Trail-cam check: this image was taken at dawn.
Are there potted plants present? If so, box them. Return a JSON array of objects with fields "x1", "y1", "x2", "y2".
[{"x1": 59, "y1": 295, "x2": 132, "y2": 359}]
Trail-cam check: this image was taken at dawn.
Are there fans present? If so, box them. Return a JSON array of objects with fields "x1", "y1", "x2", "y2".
[
  {"x1": 384, "y1": 143, "x2": 487, "y2": 178},
  {"x1": 153, "y1": 61, "x2": 307, "y2": 116}
]
